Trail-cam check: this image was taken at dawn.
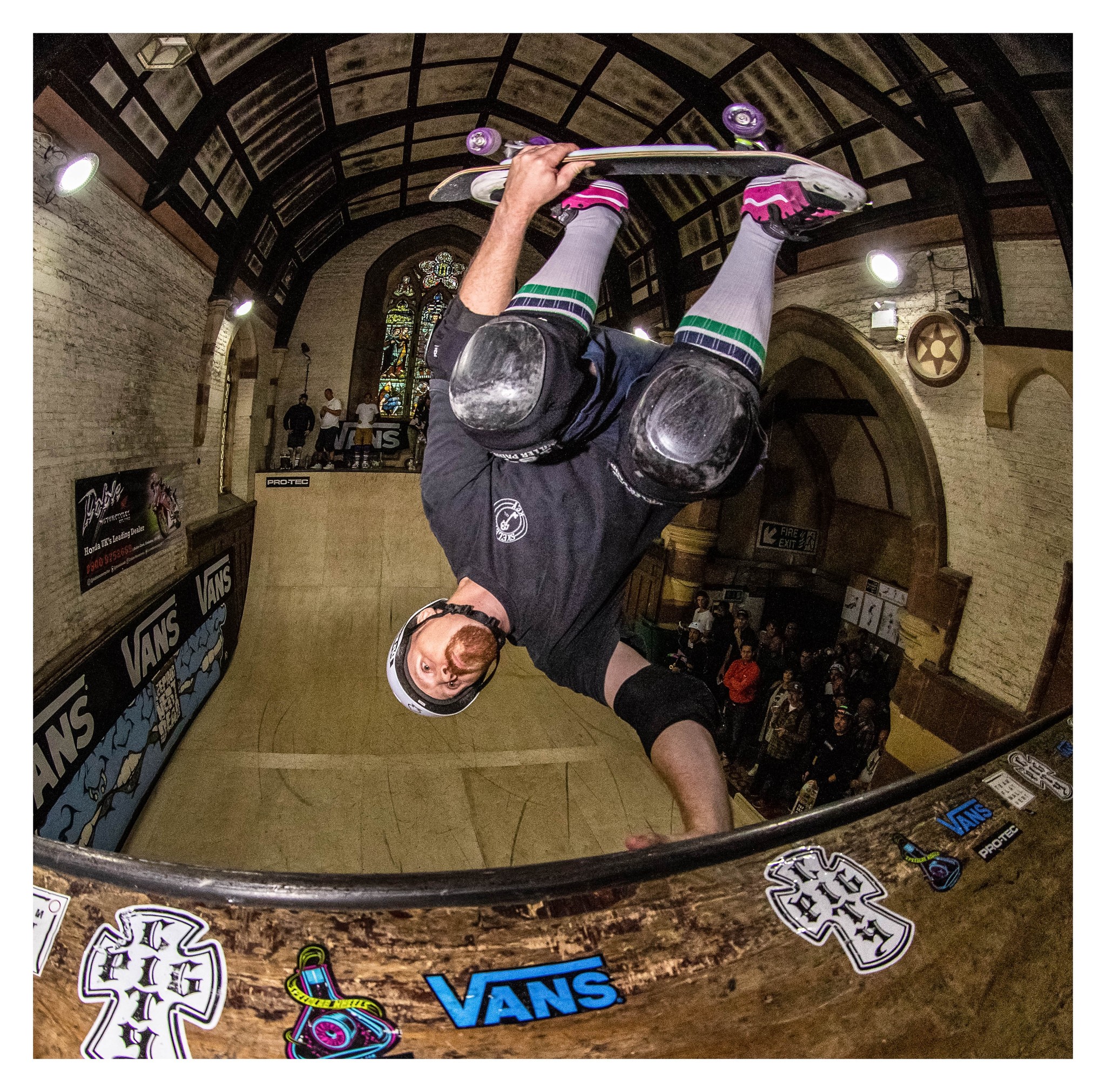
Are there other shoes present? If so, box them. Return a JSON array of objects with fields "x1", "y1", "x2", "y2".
[
  {"x1": 747, "y1": 764, "x2": 759, "y2": 776},
  {"x1": 752, "y1": 799, "x2": 769, "y2": 808}
]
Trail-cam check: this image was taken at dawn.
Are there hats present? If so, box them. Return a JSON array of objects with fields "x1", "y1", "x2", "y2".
[
  {"x1": 837, "y1": 705, "x2": 851, "y2": 717},
  {"x1": 785, "y1": 681, "x2": 802, "y2": 694}
]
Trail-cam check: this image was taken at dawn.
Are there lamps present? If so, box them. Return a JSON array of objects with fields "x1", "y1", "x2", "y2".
[
  {"x1": 869, "y1": 301, "x2": 899, "y2": 329},
  {"x1": 46, "y1": 145, "x2": 99, "y2": 198},
  {"x1": 865, "y1": 248, "x2": 908, "y2": 288},
  {"x1": 227, "y1": 297, "x2": 255, "y2": 317}
]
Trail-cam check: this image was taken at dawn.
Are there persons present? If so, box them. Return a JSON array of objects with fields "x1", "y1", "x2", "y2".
[
  {"x1": 388, "y1": 144, "x2": 868, "y2": 855},
  {"x1": 678, "y1": 590, "x2": 891, "y2": 809},
  {"x1": 282, "y1": 394, "x2": 315, "y2": 470},
  {"x1": 351, "y1": 393, "x2": 380, "y2": 469},
  {"x1": 309, "y1": 388, "x2": 342, "y2": 469}
]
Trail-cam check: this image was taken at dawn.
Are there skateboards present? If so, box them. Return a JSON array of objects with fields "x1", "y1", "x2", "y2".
[
  {"x1": 427, "y1": 103, "x2": 868, "y2": 242},
  {"x1": 280, "y1": 449, "x2": 291, "y2": 470},
  {"x1": 790, "y1": 779, "x2": 821, "y2": 816}
]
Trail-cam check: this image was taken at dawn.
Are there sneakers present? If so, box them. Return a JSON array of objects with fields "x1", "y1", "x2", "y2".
[
  {"x1": 740, "y1": 163, "x2": 869, "y2": 241},
  {"x1": 469, "y1": 170, "x2": 631, "y2": 231}
]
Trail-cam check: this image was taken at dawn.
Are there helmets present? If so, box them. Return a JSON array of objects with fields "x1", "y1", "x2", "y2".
[
  {"x1": 829, "y1": 663, "x2": 845, "y2": 677},
  {"x1": 688, "y1": 622, "x2": 705, "y2": 633},
  {"x1": 386, "y1": 597, "x2": 482, "y2": 718}
]
